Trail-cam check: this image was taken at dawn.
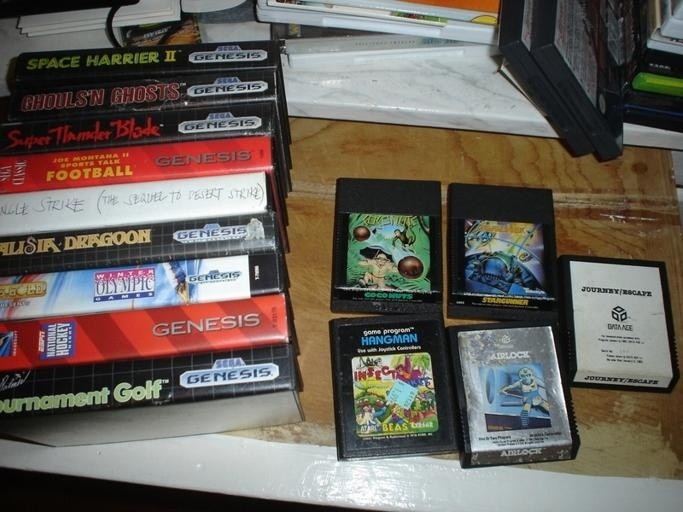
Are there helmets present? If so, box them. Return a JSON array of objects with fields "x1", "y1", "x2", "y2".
[{"x1": 518, "y1": 367, "x2": 533, "y2": 379}]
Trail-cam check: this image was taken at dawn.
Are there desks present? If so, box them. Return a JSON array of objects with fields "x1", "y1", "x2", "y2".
[{"x1": 0, "y1": 117, "x2": 683, "y2": 512}]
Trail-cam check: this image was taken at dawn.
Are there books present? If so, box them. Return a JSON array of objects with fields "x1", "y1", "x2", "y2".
[
  {"x1": 256, "y1": 0, "x2": 683, "y2": 161},
  {"x1": 329, "y1": 177, "x2": 445, "y2": 316},
  {"x1": 443, "y1": 321, "x2": 581, "y2": 469},
  {"x1": 446, "y1": 182, "x2": 559, "y2": 324},
  {"x1": 327, "y1": 316, "x2": 458, "y2": 462},
  {"x1": 1, "y1": 1, "x2": 309, "y2": 449},
  {"x1": 557, "y1": 253, "x2": 680, "y2": 393}
]
